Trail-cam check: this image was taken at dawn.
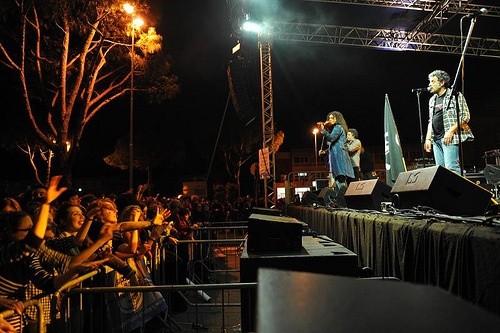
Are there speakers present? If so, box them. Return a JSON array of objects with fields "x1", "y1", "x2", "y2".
[{"x1": 240, "y1": 165, "x2": 500, "y2": 332}]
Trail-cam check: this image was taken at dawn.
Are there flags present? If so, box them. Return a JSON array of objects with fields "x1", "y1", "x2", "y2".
[{"x1": 384, "y1": 96, "x2": 405, "y2": 186}]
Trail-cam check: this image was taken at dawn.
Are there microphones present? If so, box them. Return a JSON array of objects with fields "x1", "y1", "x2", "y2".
[
  {"x1": 467, "y1": 7, "x2": 489, "y2": 17},
  {"x1": 412, "y1": 87, "x2": 431, "y2": 92}
]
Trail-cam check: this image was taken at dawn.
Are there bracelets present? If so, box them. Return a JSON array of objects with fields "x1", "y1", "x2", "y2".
[{"x1": 426, "y1": 139, "x2": 431, "y2": 141}]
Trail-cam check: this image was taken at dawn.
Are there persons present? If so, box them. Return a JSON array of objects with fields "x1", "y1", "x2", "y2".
[
  {"x1": 424, "y1": 70, "x2": 474, "y2": 175},
  {"x1": 318, "y1": 112, "x2": 355, "y2": 208},
  {"x1": 347, "y1": 130, "x2": 361, "y2": 181},
  {"x1": 0, "y1": 176, "x2": 275, "y2": 333}
]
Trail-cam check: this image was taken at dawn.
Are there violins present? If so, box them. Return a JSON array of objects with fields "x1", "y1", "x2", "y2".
[{"x1": 316, "y1": 121, "x2": 333, "y2": 131}]
